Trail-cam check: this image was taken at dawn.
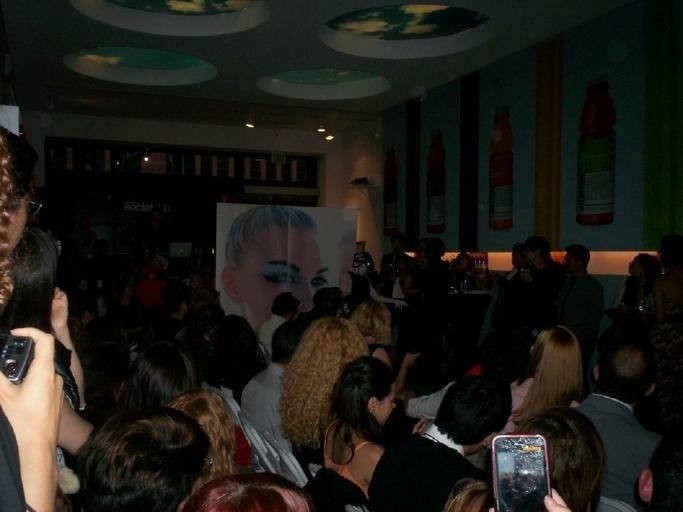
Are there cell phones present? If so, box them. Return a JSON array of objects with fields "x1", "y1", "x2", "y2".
[{"x1": 491, "y1": 434, "x2": 553, "y2": 512}]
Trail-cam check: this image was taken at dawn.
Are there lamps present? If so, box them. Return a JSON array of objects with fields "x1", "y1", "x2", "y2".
[
  {"x1": 243, "y1": 111, "x2": 256, "y2": 130},
  {"x1": 316, "y1": 119, "x2": 336, "y2": 142}
]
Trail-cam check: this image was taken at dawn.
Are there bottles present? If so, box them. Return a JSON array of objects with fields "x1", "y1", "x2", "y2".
[
  {"x1": 383, "y1": 167, "x2": 397, "y2": 238},
  {"x1": 463, "y1": 275, "x2": 468, "y2": 295},
  {"x1": 424, "y1": 130, "x2": 448, "y2": 235},
  {"x1": 473, "y1": 255, "x2": 486, "y2": 273},
  {"x1": 488, "y1": 106, "x2": 516, "y2": 229},
  {"x1": 59, "y1": 151, "x2": 298, "y2": 184},
  {"x1": 574, "y1": 83, "x2": 615, "y2": 227}
]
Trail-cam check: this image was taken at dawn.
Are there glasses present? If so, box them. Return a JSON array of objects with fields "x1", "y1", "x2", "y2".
[{"x1": 12, "y1": 194, "x2": 46, "y2": 217}]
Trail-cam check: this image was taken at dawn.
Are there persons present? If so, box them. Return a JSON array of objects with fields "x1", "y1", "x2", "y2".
[
  {"x1": 0, "y1": 122, "x2": 52, "y2": 512},
  {"x1": 221, "y1": 203, "x2": 355, "y2": 330},
  {"x1": 0, "y1": 223, "x2": 89, "y2": 498},
  {"x1": 138, "y1": 205, "x2": 175, "y2": 255}
]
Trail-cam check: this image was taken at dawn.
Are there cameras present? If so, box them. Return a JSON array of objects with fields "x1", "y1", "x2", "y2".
[{"x1": 0, "y1": 332, "x2": 34, "y2": 386}]
